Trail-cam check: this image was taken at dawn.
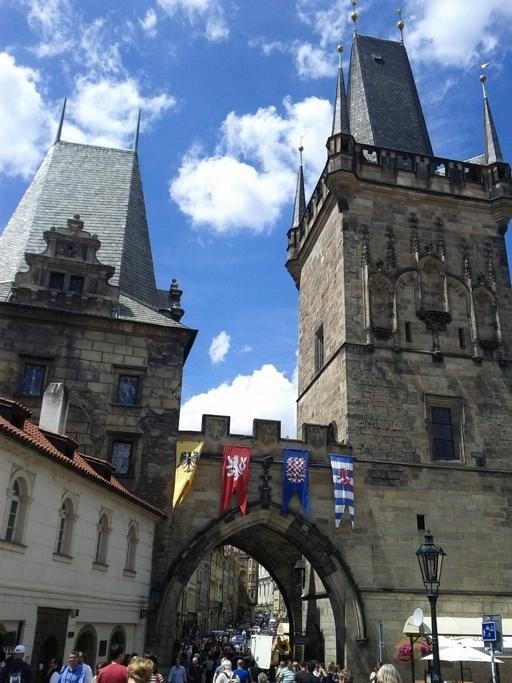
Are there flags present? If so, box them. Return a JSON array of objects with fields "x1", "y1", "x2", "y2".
[
  {"x1": 221, "y1": 442, "x2": 251, "y2": 514},
  {"x1": 281, "y1": 447, "x2": 310, "y2": 513},
  {"x1": 329, "y1": 453, "x2": 356, "y2": 529}
]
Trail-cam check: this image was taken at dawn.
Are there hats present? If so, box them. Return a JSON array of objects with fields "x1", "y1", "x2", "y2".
[{"x1": 14, "y1": 644, "x2": 26, "y2": 654}]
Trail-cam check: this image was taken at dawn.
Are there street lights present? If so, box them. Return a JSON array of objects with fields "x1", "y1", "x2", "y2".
[{"x1": 412, "y1": 525, "x2": 450, "y2": 682}]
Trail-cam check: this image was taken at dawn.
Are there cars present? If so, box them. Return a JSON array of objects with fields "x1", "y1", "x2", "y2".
[{"x1": 208, "y1": 612, "x2": 278, "y2": 647}]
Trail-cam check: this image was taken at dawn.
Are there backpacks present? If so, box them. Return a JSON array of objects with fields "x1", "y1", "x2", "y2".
[{"x1": 220, "y1": 672, "x2": 240, "y2": 683}]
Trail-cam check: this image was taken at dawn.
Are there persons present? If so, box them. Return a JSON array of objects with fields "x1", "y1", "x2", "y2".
[{"x1": 0, "y1": 644, "x2": 33, "y2": 682}]
